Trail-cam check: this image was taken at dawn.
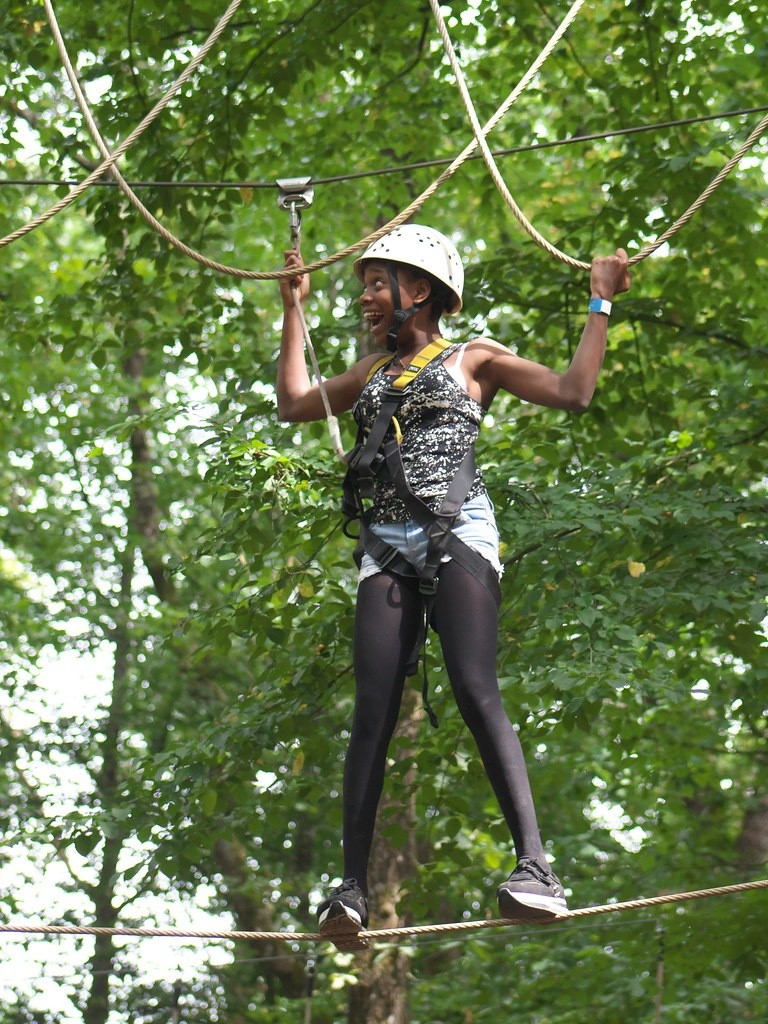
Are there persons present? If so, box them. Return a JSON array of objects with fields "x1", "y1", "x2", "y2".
[{"x1": 279, "y1": 224, "x2": 631, "y2": 951}]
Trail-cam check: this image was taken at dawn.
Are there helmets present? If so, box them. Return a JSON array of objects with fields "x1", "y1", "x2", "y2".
[{"x1": 353, "y1": 224, "x2": 465, "y2": 316}]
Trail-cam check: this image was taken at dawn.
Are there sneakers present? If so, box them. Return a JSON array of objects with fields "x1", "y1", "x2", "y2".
[
  {"x1": 318, "y1": 878, "x2": 369, "y2": 952},
  {"x1": 497, "y1": 857, "x2": 569, "y2": 920}
]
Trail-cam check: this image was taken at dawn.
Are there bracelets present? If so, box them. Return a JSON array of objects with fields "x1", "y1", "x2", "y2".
[{"x1": 589, "y1": 297, "x2": 613, "y2": 316}]
